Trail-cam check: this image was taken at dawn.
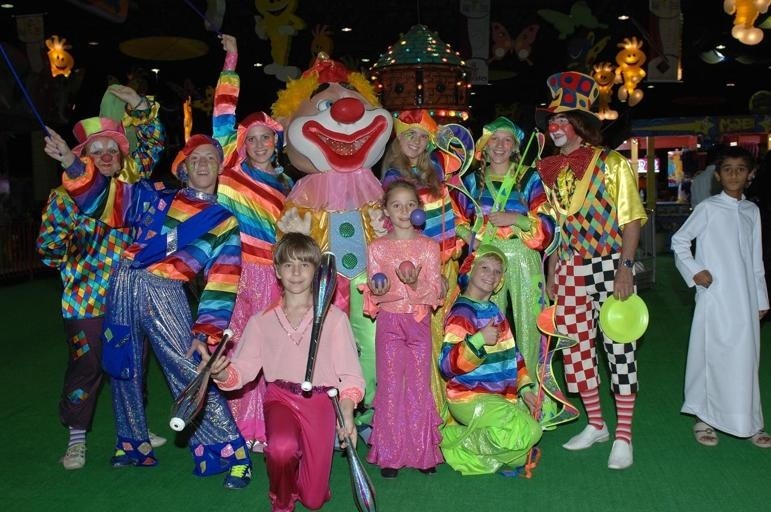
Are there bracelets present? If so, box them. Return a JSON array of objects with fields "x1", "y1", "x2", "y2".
[
  {"x1": 134, "y1": 98, "x2": 144, "y2": 110},
  {"x1": 618, "y1": 257, "x2": 634, "y2": 268}
]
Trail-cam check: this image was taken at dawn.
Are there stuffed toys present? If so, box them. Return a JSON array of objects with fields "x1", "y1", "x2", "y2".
[{"x1": 269, "y1": 52, "x2": 394, "y2": 450}]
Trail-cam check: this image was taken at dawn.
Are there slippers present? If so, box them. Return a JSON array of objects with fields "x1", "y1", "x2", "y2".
[
  {"x1": 691, "y1": 422, "x2": 720, "y2": 446},
  {"x1": 751, "y1": 429, "x2": 771, "y2": 448}
]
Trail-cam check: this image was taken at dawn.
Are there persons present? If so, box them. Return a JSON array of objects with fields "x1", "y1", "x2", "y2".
[
  {"x1": 532, "y1": 108, "x2": 650, "y2": 470},
  {"x1": 688, "y1": 145, "x2": 771, "y2": 321},
  {"x1": 450, "y1": 115, "x2": 562, "y2": 433},
  {"x1": 209, "y1": 232, "x2": 367, "y2": 512},
  {"x1": 211, "y1": 32, "x2": 298, "y2": 451},
  {"x1": 670, "y1": 146, "x2": 771, "y2": 448},
  {"x1": 438, "y1": 248, "x2": 543, "y2": 479},
  {"x1": 357, "y1": 179, "x2": 447, "y2": 477},
  {"x1": 35, "y1": 81, "x2": 168, "y2": 470},
  {"x1": 43, "y1": 123, "x2": 252, "y2": 488},
  {"x1": 380, "y1": 106, "x2": 463, "y2": 426}
]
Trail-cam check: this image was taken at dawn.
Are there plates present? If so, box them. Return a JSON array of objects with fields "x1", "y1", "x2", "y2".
[{"x1": 600, "y1": 293, "x2": 650, "y2": 343}]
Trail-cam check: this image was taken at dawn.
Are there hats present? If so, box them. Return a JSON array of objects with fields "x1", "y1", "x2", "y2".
[
  {"x1": 533, "y1": 70, "x2": 603, "y2": 133},
  {"x1": 70, "y1": 116, "x2": 130, "y2": 159},
  {"x1": 171, "y1": 134, "x2": 223, "y2": 181},
  {"x1": 457, "y1": 244, "x2": 509, "y2": 295},
  {"x1": 474, "y1": 116, "x2": 525, "y2": 163},
  {"x1": 236, "y1": 111, "x2": 284, "y2": 158},
  {"x1": 392, "y1": 108, "x2": 438, "y2": 151}
]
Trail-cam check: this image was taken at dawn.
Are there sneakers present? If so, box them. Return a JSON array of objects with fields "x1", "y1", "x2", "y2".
[
  {"x1": 607, "y1": 439, "x2": 634, "y2": 470},
  {"x1": 147, "y1": 430, "x2": 166, "y2": 447},
  {"x1": 564, "y1": 420, "x2": 610, "y2": 450},
  {"x1": 64, "y1": 442, "x2": 86, "y2": 470},
  {"x1": 223, "y1": 463, "x2": 252, "y2": 489}
]
